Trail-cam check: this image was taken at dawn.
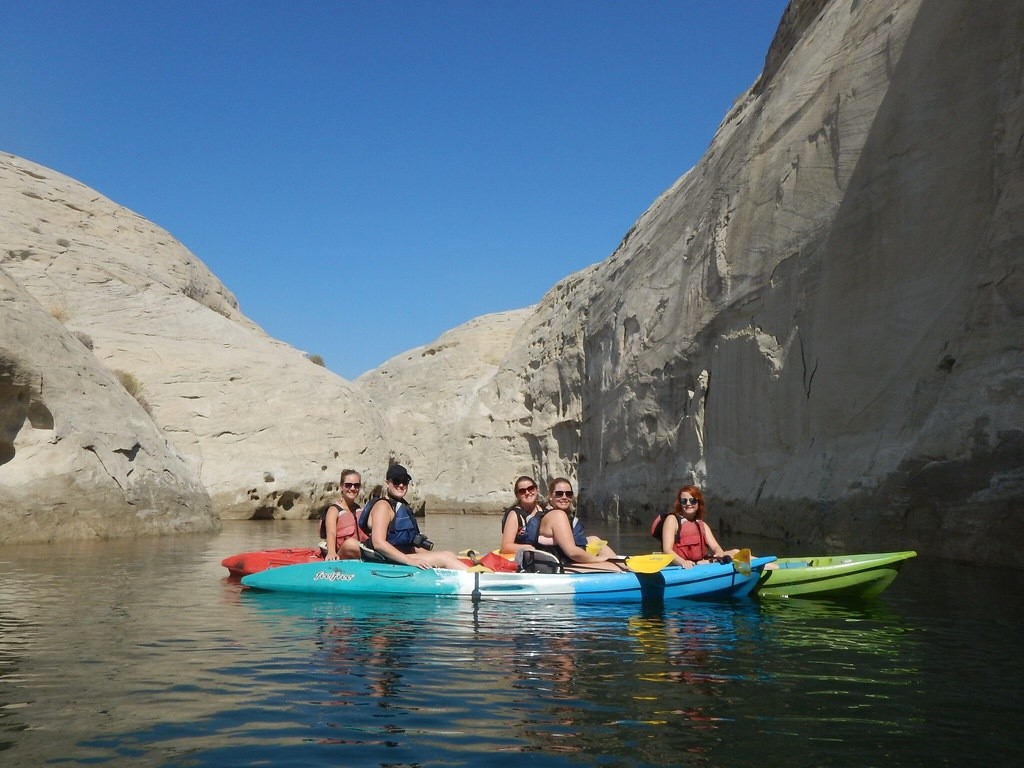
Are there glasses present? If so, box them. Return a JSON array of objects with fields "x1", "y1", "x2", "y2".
[
  {"x1": 555, "y1": 491, "x2": 573, "y2": 498},
  {"x1": 388, "y1": 478, "x2": 410, "y2": 486},
  {"x1": 679, "y1": 498, "x2": 697, "y2": 505},
  {"x1": 517, "y1": 485, "x2": 536, "y2": 495},
  {"x1": 343, "y1": 483, "x2": 360, "y2": 489}
]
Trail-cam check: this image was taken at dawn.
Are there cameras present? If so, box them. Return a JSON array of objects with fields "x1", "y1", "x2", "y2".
[{"x1": 412, "y1": 533, "x2": 434, "y2": 551}]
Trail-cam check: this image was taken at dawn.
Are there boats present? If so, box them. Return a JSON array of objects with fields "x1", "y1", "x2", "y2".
[
  {"x1": 237, "y1": 556, "x2": 778, "y2": 604},
  {"x1": 218, "y1": 551, "x2": 519, "y2": 578},
  {"x1": 753, "y1": 551, "x2": 915, "y2": 597}
]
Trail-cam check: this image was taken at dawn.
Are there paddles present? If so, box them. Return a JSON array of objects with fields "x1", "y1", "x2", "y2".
[
  {"x1": 455, "y1": 544, "x2": 493, "y2": 575},
  {"x1": 706, "y1": 546, "x2": 757, "y2": 580},
  {"x1": 586, "y1": 538, "x2": 609, "y2": 557},
  {"x1": 604, "y1": 551, "x2": 678, "y2": 576}
]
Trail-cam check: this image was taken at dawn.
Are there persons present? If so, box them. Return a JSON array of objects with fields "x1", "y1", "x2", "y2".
[
  {"x1": 501, "y1": 476, "x2": 546, "y2": 554},
  {"x1": 534, "y1": 477, "x2": 635, "y2": 573},
  {"x1": 662, "y1": 485, "x2": 758, "y2": 569},
  {"x1": 324, "y1": 469, "x2": 370, "y2": 561},
  {"x1": 367, "y1": 465, "x2": 471, "y2": 570}
]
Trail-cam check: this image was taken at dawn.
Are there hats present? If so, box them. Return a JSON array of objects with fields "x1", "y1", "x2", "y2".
[{"x1": 386, "y1": 465, "x2": 412, "y2": 480}]
